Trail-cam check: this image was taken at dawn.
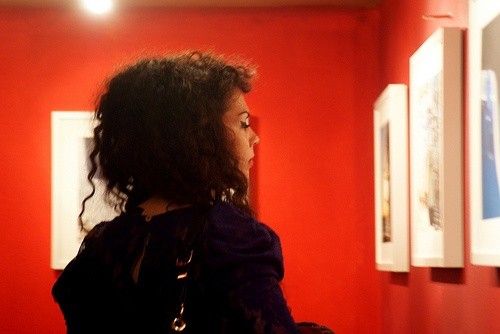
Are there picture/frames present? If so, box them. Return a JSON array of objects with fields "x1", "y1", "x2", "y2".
[
  {"x1": 410, "y1": 27, "x2": 463, "y2": 268},
  {"x1": 372, "y1": 83, "x2": 410, "y2": 273},
  {"x1": 51, "y1": 110, "x2": 249, "y2": 270},
  {"x1": 469, "y1": 0, "x2": 499, "y2": 269}
]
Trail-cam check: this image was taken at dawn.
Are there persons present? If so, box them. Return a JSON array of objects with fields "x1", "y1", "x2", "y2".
[{"x1": 51, "y1": 49, "x2": 338, "y2": 334}]
